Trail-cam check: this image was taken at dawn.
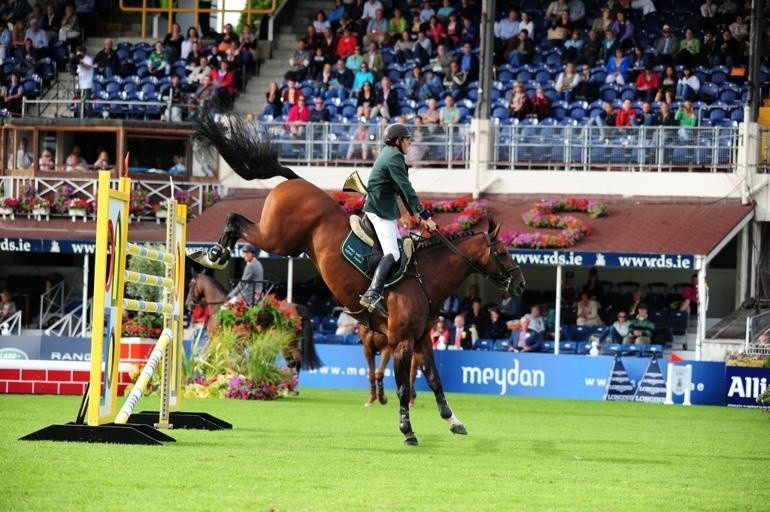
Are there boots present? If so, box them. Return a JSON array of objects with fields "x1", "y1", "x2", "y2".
[{"x1": 359, "y1": 253, "x2": 396, "y2": 318}]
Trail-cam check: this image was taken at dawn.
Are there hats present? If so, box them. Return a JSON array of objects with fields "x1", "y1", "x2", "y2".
[
  {"x1": 637, "y1": 303, "x2": 647, "y2": 309},
  {"x1": 663, "y1": 25, "x2": 671, "y2": 31}
]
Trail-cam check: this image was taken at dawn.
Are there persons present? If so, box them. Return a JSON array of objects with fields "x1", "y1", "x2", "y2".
[
  {"x1": 754, "y1": 335, "x2": 770, "y2": 353},
  {"x1": 334, "y1": 268, "x2": 696, "y2": 354},
  {"x1": 169, "y1": 152, "x2": 187, "y2": 175},
  {"x1": 220, "y1": 242, "x2": 264, "y2": 310},
  {"x1": 0, "y1": 291, "x2": 17, "y2": 329},
  {"x1": 0, "y1": 1, "x2": 85, "y2": 115},
  {"x1": 258, "y1": 0, "x2": 769, "y2": 161},
  {"x1": 5, "y1": 136, "x2": 111, "y2": 173},
  {"x1": 361, "y1": 122, "x2": 439, "y2": 319},
  {"x1": 70, "y1": 22, "x2": 261, "y2": 119}
]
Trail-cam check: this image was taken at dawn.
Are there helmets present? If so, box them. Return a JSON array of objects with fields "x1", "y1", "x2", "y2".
[
  {"x1": 384, "y1": 123, "x2": 411, "y2": 143},
  {"x1": 242, "y1": 245, "x2": 256, "y2": 252}
]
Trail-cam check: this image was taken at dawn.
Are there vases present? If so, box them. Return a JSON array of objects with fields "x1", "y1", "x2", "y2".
[{"x1": 0, "y1": 206, "x2": 173, "y2": 226}]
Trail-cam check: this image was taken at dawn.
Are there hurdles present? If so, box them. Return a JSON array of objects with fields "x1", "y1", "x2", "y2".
[{"x1": 15, "y1": 171, "x2": 233, "y2": 445}]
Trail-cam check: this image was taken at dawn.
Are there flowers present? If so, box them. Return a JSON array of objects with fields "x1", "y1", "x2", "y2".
[
  {"x1": 0, "y1": 183, "x2": 221, "y2": 215},
  {"x1": 499, "y1": 198, "x2": 610, "y2": 248},
  {"x1": 400, "y1": 199, "x2": 490, "y2": 237}
]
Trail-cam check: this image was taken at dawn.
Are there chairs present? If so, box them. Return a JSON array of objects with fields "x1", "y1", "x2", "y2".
[{"x1": 0, "y1": 28, "x2": 192, "y2": 119}]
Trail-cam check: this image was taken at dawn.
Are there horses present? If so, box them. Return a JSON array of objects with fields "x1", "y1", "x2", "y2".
[
  {"x1": 184, "y1": 266, "x2": 326, "y2": 397},
  {"x1": 358, "y1": 322, "x2": 417, "y2": 407},
  {"x1": 189, "y1": 100, "x2": 526, "y2": 447}
]
